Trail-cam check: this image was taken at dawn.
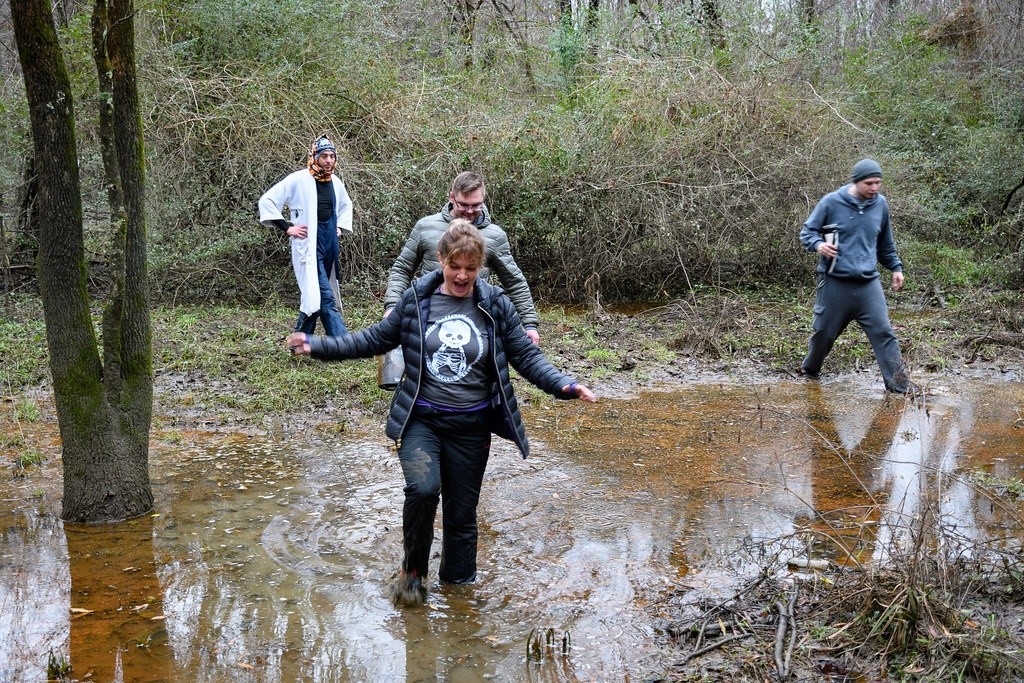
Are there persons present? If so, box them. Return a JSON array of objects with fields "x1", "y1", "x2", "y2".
[
  {"x1": 284, "y1": 221, "x2": 597, "y2": 605},
  {"x1": 257, "y1": 139, "x2": 353, "y2": 338},
  {"x1": 383, "y1": 172, "x2": 540, "y2": 345},
  {"x1": 800, "y1": 158, "x2": 911, "y2": 394}
]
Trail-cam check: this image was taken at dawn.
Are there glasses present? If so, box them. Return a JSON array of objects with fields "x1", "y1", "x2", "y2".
[{"x1": 453, "y1": 196, "x2": 484, "y2": 211}]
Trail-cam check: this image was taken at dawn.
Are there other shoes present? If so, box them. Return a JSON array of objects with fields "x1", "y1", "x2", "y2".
[{"x1": 399, "y1": 564, "x2": 424, "y2": 606}]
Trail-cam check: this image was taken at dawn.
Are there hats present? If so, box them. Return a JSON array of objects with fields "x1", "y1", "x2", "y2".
[
  {"x1": 851, "y1": 159, "x2": 883, "y2": 184},
  {"x1": 314, "y1": 136, "x2": 336, "y2": 160}
]
